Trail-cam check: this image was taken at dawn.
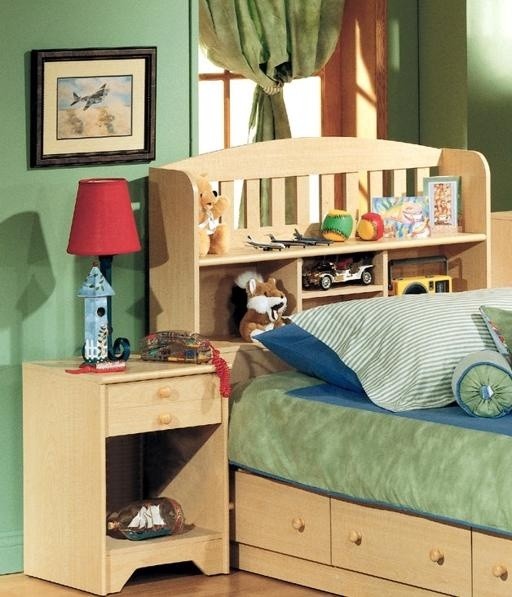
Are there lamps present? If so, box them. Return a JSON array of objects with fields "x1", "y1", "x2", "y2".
[{"x1": 65, "y1": 176, "x2": 143, "y2": 363}]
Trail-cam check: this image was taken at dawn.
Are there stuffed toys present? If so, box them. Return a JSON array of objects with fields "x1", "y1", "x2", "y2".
[
  {"x1": 230, "y1": 270, "x2": 264, "y2": 338},
  {"x1": 239, "y1": 277, "x2": 288, "y2": 343},
  {"x1": 195, "y1": 176, "x2": 230, "y2": 259}
]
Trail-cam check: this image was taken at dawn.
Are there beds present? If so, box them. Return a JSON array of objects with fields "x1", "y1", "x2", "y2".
[{"x1": 146, "y1": 135, "x2": 512, "y2": 595}]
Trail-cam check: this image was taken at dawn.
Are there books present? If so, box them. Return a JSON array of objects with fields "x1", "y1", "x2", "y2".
[{"x1": 478, "y1": 305, "x2": 512, "y2": 370}]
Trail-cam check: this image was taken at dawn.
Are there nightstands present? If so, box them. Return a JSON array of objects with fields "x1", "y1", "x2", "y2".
[{"x1": 19, "y1": 356, "x2": 233, "y2": 595}]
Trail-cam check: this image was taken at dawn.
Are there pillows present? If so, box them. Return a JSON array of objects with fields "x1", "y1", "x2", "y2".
[{"x1": 250, "y1": 284, "x2": 512, "y2": 414}]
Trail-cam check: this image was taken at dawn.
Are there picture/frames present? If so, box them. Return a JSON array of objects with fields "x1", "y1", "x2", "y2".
[{"x1": 27, "y1": 44, "x2": 157, "y2": 169}]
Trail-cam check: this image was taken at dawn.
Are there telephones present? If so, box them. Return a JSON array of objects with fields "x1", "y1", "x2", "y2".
[{"x1": 138, "y1": 330, "x2": 211, "y2": 363}]
